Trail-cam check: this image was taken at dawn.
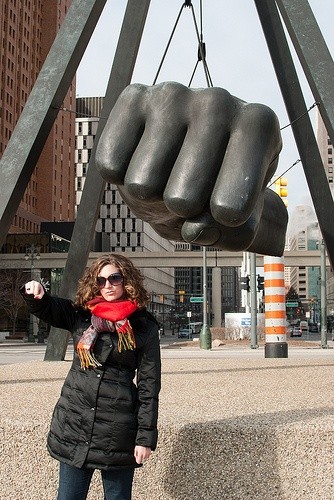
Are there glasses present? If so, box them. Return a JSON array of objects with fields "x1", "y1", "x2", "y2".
[{"x1": 94, "y1": 272, "x2": 124, "y2": 288}]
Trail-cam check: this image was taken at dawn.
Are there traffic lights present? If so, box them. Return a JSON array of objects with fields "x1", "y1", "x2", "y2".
[
  {"x1": 275, "y1": 175, "x2": 288, "y2": 208},
  {"x1": 240, "y1": 276, "x2": 250, "y2": 292},
  {"x1": 310, "y1": 298, "x2": 314, "y2": 305},
  {"x1": 257, "y1": 274, "x2": 264, "y2": 291}
]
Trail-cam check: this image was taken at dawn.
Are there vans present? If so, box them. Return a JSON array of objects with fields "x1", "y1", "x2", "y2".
[{"x1": 300, "y1": 320, "x2": 309, "y2": 330}]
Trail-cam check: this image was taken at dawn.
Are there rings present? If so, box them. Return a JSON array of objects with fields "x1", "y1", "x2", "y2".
[{"x1": 27, "y1": 288, "x2": 29, "y2": 290}]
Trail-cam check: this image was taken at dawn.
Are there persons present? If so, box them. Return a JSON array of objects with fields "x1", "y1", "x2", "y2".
[{"x1": 18, "y1": 254, "x2": 161, "y2": 500}]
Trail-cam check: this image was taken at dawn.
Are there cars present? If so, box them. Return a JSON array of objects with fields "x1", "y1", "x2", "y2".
[
  {"x1": 178, "y1": 327, "x2": 194, "y2": 338},
  {"x1": 310, "y1": 326, "x2": 318, "y2": 332},
  {"x1": 288, "y1": 326, "x2": 302, "y2": 337}
]
